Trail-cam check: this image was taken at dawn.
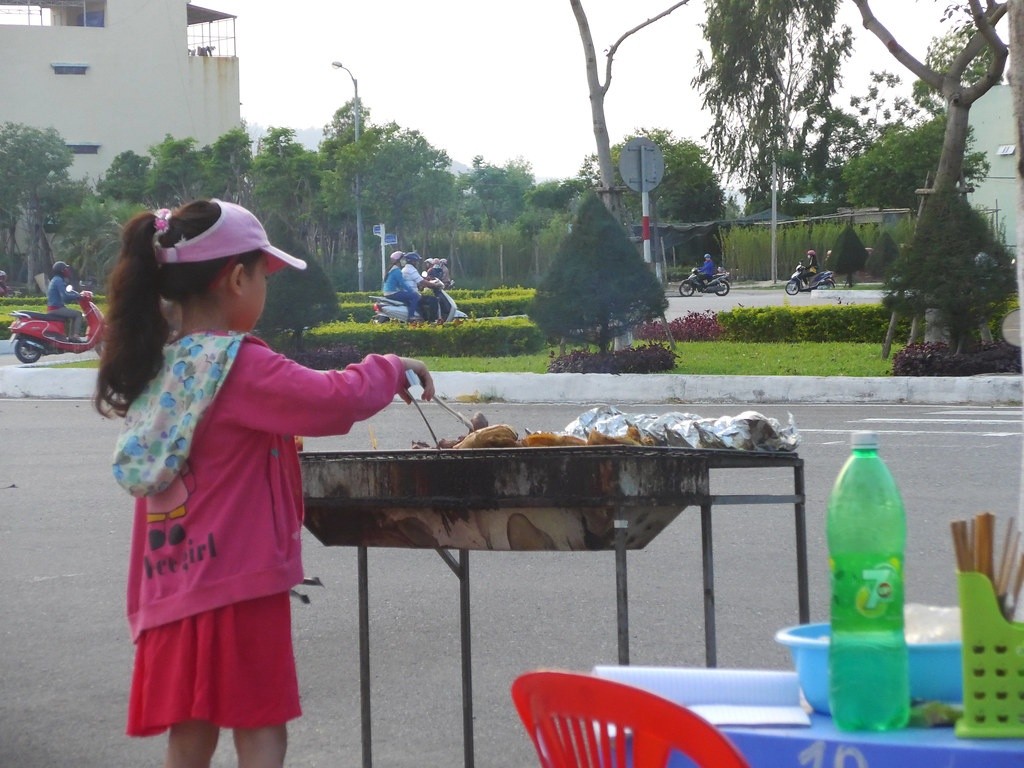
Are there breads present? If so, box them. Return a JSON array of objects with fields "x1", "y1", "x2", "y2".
[{"x1": 451, "y1": 423, "x2": 642, "y2": 448}]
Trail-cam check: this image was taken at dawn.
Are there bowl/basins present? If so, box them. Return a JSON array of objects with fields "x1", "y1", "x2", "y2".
[{"x1": 773, "y1": 620, "x2": 964, "y2": 719}]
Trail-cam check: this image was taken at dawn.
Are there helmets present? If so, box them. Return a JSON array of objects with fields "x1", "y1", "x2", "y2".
[
  {"x1": 425, "y1": 258, "x2": 433, "y2": 264},
  {"x1": 440, "y1": 258, "x2": 447, "y2": 264},
  {"x1": 406, "y1": 252, "x2": 422, "y2": 260},
  {"x1": 705, "y1": 254, "x2": 710, "y2": 258},
  {"x1": 390, "y1": 251, "x2": 405, "y2": 263},
  {"x1": 808, "y1": 250, "x2": 815, "y2": 255},
  {"x1": 53, "y1": 262, "x2": 68, "y2": 271}
]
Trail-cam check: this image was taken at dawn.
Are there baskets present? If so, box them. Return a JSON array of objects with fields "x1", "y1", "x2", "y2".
[{"x1": 953, "y1": 572, "x2": 1024, "y2": 737}]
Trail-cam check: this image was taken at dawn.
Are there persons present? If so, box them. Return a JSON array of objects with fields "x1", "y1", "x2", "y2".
[
  {"x1": 424, "y1": 258, "x2": 450, "y2": 285},
  {"x1": 383, "y1": 251, "x2": 438, "y2": 322},
  {"x1": 47, "y1": 261, "x2": 83, "y2": 343},
  {"x1": 698, "y1": 254, "x2": 714, "y2": 291},
  {"x1": 92, "y1": 198, "x2": 435, "y2": 768},
  {"x1": 801, "y1": 249, "x2": 817, "y2": 287}
]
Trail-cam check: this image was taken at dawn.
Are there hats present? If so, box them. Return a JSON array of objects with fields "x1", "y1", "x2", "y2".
[
  {"x1": 433, "y1": 258, "x2": 439, "y2": 264},
  {"x1": 155, "y1": 199, "x2": 307, "y2": 275}
]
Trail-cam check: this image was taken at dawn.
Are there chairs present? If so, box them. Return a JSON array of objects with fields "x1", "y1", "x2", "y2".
[{"x1": 511, "y1": 671, "x2": 752, "y2": 768}]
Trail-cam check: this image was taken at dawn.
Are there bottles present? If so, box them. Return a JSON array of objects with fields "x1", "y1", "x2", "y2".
[{"x1": 823, "y1": 431, "x2": 910, "y2": 733}]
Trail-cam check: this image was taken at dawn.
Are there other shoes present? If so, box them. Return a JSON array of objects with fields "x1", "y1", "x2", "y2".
[
  {"x1": 408, "y1": 315, "x2": 422, "y2": 321},
  {"x1": 73, "y1": 336, "x2": 87, "y2": 343}
]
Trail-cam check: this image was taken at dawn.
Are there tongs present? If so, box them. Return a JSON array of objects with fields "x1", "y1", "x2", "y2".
[
  {"x1": 403, "y1": 385, "x2": 475, "y2": 449},
  {"x1": 289, "y1": 576, "x2": 324, "y2": 604}
]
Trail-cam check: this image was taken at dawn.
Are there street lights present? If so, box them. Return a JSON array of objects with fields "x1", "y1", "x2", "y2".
[{"x1": 331, "y1": 61, "x2": 365, "y2": 292}]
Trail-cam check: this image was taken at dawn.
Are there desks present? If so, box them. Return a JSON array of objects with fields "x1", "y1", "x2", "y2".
[{"x1": 603, "y1": 709, "x2": 1024, "y2": 768}]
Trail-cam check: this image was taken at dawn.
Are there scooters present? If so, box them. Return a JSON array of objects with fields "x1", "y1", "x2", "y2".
[
  {"x1": 678, "y1": 267, "x2": 731, "y2": 297},
  {"x1": 368, "y1": 260, "x2": 470, "y2": 323},
  {"x1": 785, "y1": 260, "x2": 837, "y2": 295},
  {"x1": 7, "y1": 285, "x2": 109, "y2": 364}
]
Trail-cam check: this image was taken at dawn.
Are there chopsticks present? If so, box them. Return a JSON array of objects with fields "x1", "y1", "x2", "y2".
[{"x1": 950, "y1": 511, "x2": 1024, "y2": 623}]
